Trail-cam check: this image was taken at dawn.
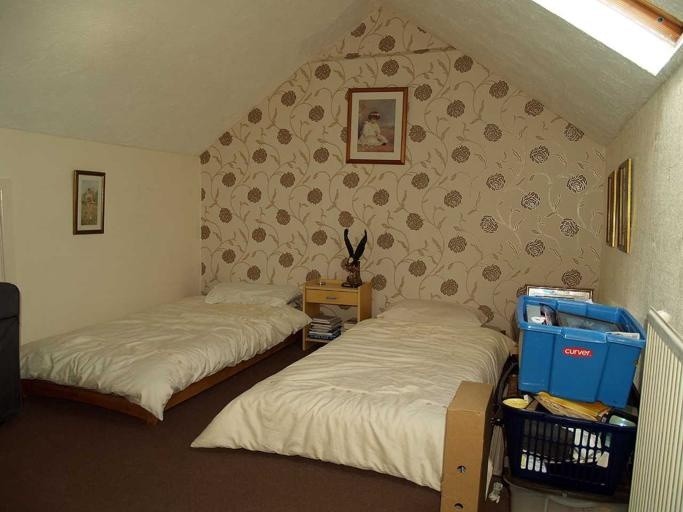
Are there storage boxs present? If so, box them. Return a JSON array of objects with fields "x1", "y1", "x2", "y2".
[
  {"x1": 514, "y1": 292, "x2": 646, "y2": 407},
  {"x1": 499, "y1": 474, "x2": 624, "y2": 512}
]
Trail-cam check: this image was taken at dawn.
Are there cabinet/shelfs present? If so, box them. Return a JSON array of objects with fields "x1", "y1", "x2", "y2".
[{"x1": 0, "y1": 279, "x2": 23, "y2": 425}]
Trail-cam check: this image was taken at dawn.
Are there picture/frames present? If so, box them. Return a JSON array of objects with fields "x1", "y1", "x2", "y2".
[
  {"x1": 617, "y1": 157, "x2": 631, "y2": 255},
  {"x1": 73, "y1": 169, "x2": 104, "y2": 235},
  {"x1": 348, "y1": 87, "x2": 408, "y2": 163},
  {"x1": 605, "y1": 170, "x2": 617, "y2": 248}
]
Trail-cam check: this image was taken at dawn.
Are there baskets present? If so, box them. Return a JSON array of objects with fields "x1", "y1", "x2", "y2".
[{"x1": 496, "y1": 363, "x2": 637, "y2": 503}]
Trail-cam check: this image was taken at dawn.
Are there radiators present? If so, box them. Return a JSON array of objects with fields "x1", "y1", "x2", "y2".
[{"x1": 627, "y1": 307, "x2": 682, "y2": 512}]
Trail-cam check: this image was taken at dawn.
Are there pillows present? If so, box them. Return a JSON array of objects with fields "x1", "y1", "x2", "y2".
[
  {"x1": 376, "y1": 299, "x2": 488, "y2": 327},
  {"x1": 205, "y1": 282, "x2": 301, "y2": 307}
]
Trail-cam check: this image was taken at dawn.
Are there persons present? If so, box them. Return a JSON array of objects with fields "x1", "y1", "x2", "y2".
[
  {"x1": 357, "y1": 111, "x2": 388, "y2": 147},
  {"x1": 307, "y1": 315, "x2": 358, "y2": 340}
]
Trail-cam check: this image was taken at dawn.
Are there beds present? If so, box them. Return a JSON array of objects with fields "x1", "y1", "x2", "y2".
[
  {"x1": 18, "y1": 297, "x2": 311, "y2": 427},
  {"x1": 191, "y1": 319, "x2": 518, "y2": 492}
]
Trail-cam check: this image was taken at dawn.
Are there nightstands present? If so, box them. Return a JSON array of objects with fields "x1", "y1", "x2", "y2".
[{"x1": 302, "y1": 280, "x2": 371, "y2": 351}]
